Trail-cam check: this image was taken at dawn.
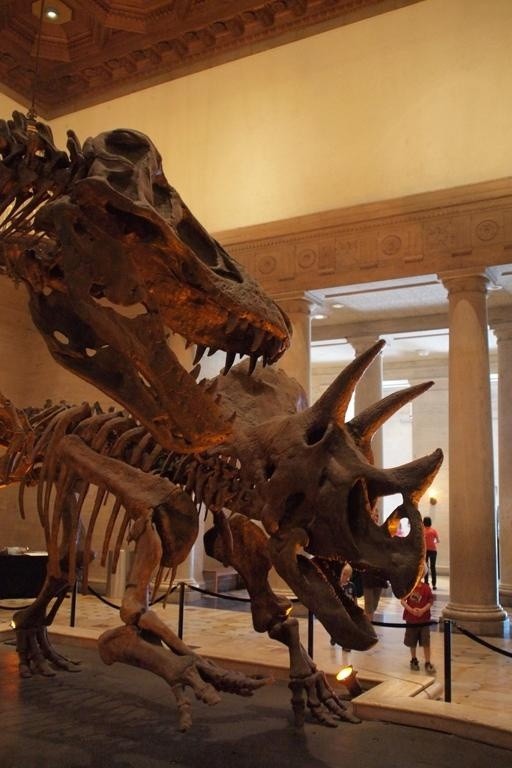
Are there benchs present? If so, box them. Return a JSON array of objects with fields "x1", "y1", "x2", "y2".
[{"x1": 202, "y1": 567, "x2": 242, "y2": 595}]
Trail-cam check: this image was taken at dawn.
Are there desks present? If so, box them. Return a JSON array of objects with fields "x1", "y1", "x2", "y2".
[{"x1": 0, "y1": 549, "x2": 95, "y2": 601}]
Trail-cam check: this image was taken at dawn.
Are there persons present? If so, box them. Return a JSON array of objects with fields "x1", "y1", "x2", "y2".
[
  {"x1": 330, "y1": 561, "x2": 363, "y2": 653},
  {"x1": 401, "y1": 579, "x2": 436, "y2": 674},
  {"x1": 423, "y1": 517, "x2": 440, "y2": 590}
]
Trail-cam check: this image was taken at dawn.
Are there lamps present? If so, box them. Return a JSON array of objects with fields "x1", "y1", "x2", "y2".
[{"x1": 427, "y1": 489, "x2": 437, "y2": 507}]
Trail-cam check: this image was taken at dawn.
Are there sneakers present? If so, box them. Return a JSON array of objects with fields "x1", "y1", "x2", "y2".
[
  {"x1": 424, "y1": 662, "x2": 437, "y2": 675},
  {"x1": 410, "y1": 658, "x2": 420, "y2": 671},
  {"x1": 433, "y1": 587, "x2": 437, "y2": 590}
]
